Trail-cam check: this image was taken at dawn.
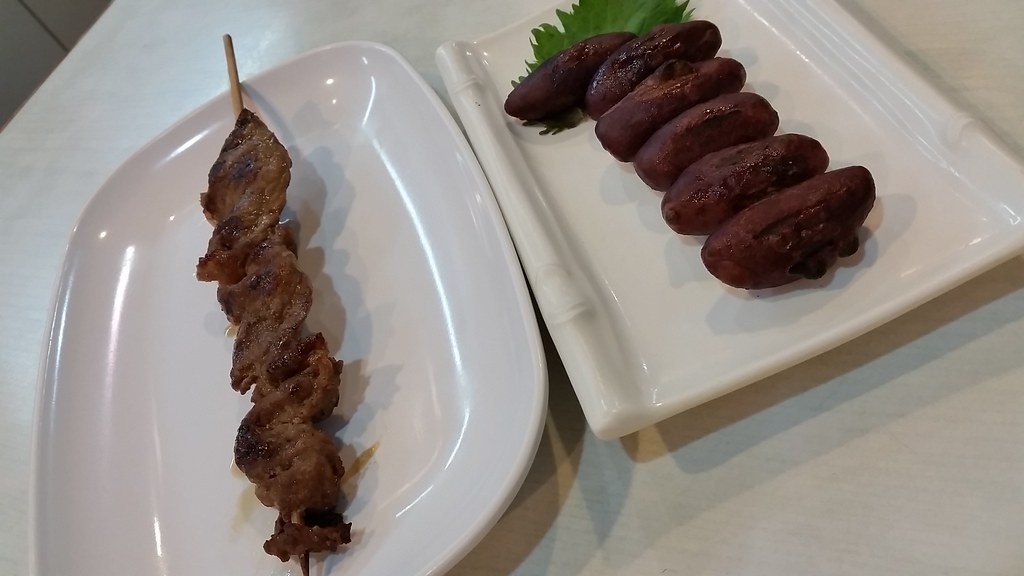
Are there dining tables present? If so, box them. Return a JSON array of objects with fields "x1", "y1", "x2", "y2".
[{"x1": 0, "y1": 0, "x2": 1024, "y2": 576}]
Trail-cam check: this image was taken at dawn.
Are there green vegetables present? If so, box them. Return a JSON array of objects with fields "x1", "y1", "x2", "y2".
[{"x1": 511, "y1": 0, "x2": 695, "y2": 137}]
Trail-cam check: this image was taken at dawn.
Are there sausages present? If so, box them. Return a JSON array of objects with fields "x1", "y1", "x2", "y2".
[{"x1": 504, "y1": 21, "x2": 876, "y2": 289}]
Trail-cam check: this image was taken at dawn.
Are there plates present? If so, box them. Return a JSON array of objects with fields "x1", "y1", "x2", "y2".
[
  {"x1": 436, "y1": 0, "x2": 1023, "y2": 439},
  {"x1": 30, "y1": 40, "x2": 547, "y2": 575}
]
